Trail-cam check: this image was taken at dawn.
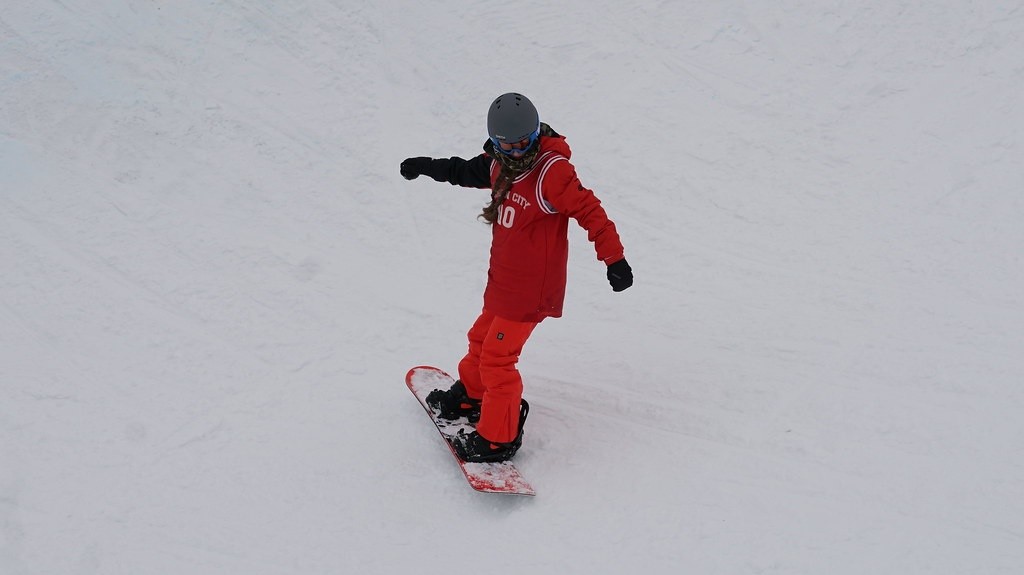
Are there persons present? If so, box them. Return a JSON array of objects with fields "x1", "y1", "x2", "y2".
[{"x1": 398, "y1": 92, "x2": 634, "y2": 462}]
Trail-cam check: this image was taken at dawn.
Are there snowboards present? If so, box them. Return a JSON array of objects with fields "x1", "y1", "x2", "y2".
[{"x1": 405, "y1": 365, "x2": 537, "y2": 496}]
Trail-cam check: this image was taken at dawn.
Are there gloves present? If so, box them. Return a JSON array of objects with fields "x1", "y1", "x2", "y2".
[
  {"x1": 607, "y1": 259, "x2": 633, "y2": 292},
  {"x1": 400, "y1": 157, "x2": 432, "y2": 181}
]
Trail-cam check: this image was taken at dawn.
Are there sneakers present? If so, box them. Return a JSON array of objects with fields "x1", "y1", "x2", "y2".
[
  {"x1": 454, "y1": 430, "x2": 515, "y2": 463},
  {"x1": 425, "y1": 380, "x2": 482, "y2": 422}
]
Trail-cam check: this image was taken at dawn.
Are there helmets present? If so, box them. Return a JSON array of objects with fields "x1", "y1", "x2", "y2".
[{"x1": 487, "y1": 93, "x2": 538, "y2": 140}]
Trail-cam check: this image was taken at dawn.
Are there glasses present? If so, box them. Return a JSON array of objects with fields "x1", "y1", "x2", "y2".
[{"x1": 490, "y1": 118, "x2": 541, "y2": 155}]
